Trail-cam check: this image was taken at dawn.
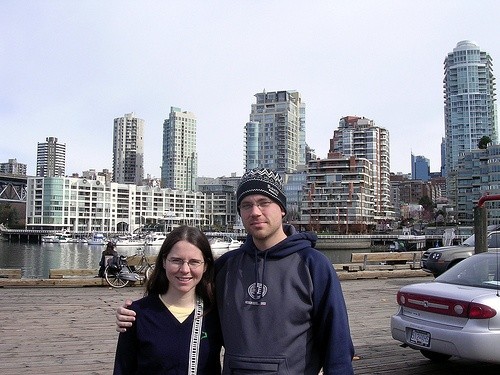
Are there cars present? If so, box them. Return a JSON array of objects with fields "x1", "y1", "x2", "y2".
[{"x1": 391, "y1": 252, "x2": 500, "y2": 365}]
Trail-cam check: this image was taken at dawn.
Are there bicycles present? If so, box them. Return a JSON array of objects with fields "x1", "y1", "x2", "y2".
[{"x1": 104, "y1": 241, "x2": 157, "y2": 288}]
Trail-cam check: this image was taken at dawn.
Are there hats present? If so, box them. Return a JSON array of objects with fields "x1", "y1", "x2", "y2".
[{"x1": 236, "y1": 168, "x2": 287, "y2": 217}]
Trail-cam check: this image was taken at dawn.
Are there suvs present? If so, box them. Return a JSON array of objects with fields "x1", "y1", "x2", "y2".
[{"x1": 419, "y1": 231, "x2": 500, "y2": 279}]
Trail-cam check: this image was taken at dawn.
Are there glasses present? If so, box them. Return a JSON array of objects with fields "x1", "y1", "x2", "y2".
[
  {"x1": 236, "y1": 200, "x2": 277, "y2": 209},
  {"x1": 164, "y1": 257, "x2": 206, "y2": 269}
]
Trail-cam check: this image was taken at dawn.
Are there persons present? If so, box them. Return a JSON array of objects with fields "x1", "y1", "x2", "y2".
[
  {"x1": 112, "y1": 224, "x2": 223, "y2": 375},
  {"x1": 116, "y1": 166, "x2": 355, "y2": 375},
  {"x1": 98, "y1": 242, "x2": 118, "y2": 277}
]
